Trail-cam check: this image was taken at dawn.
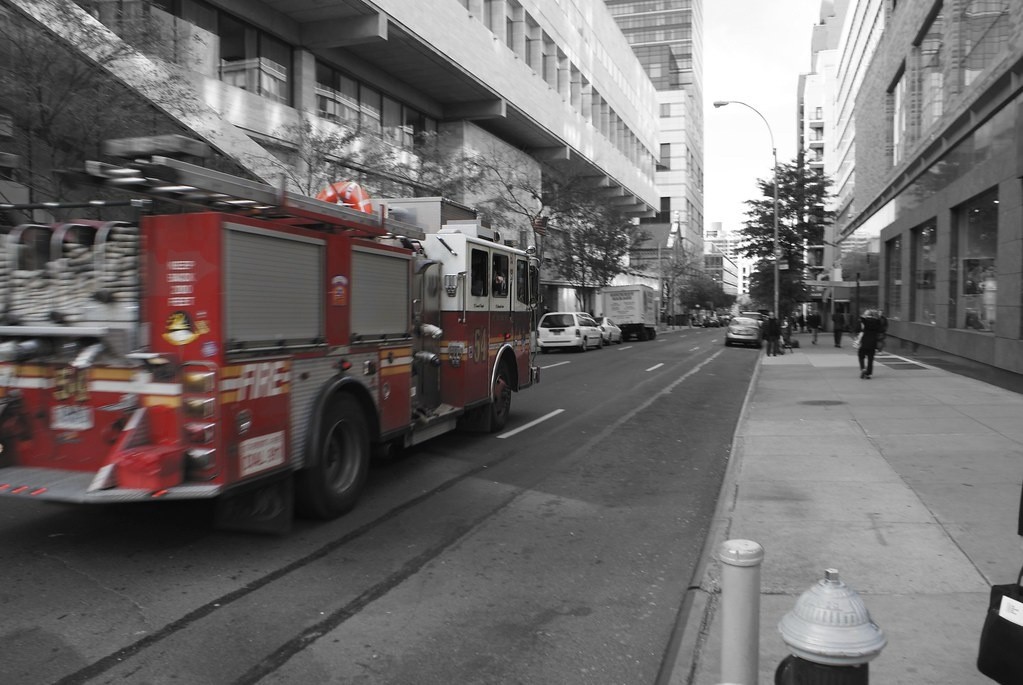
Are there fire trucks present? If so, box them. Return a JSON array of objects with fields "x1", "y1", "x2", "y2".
[{"x1": 1, "y1": 135, "x2": 542, "y2": 527}]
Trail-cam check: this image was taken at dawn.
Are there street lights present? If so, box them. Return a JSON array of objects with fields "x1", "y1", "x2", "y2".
[{"x1": 712, "y1": 100, "x2": 780, "y2": 319}]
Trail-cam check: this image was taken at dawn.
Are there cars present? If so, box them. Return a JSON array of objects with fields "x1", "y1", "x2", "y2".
[
  {"x1": 537, "y1": 311, "x2": 604, "y2": 353},
  {"x1": 694, "y1": 308, "x2": 769, "y2": 328},
  {"x1": 591, "y1": 316, "x2": 624, "y2": 346},
  {"x1": 726, "y1": 317, "x2": 761, "y2": 348}
]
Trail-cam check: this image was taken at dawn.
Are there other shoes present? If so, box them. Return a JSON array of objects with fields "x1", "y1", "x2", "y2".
[
  {"x1": 860, "y1": 369, "x2": 867, "y2": 378},
  {"x1": 865, "y1": 375, "x2": 872, "y2": 378}
]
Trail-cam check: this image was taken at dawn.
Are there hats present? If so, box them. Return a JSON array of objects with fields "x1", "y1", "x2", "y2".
[{"x1": 860, "y1": 310, "x2": 876, "y2": 318}]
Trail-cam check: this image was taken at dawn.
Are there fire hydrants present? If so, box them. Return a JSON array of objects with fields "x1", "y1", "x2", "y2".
[{"x1": 773, "y1": 566, "x2": 889, "y2": 685}]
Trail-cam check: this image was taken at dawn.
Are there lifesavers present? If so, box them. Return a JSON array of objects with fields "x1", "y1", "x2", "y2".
[{"x1": 315, "y1": 181, "x2": 372, "y2": 214}]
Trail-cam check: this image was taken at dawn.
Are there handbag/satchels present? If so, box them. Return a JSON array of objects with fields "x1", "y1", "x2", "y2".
[
  {"x1": 976, "y1": 568, "x2": 1023, "y2": 685},
  {"x1": 852, "y1": 332, "x2": 864, "y2": 351}
]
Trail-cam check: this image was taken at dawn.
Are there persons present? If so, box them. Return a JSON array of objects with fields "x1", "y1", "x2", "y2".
[
  {"x1": 764, "y1": 308, "x2": 845, "y2": 357},
  {"x1": 855, "y1": 308, "x2": 888, "y2": 379}
]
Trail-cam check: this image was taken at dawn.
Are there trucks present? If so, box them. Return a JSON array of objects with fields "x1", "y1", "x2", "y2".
[{"x1": 600, "y1": 284, "x2": 658, "y2": 342}]
